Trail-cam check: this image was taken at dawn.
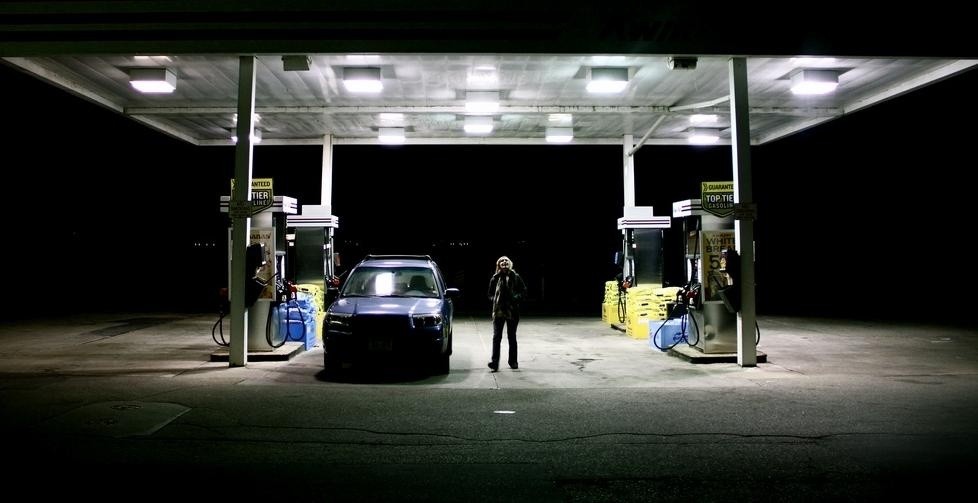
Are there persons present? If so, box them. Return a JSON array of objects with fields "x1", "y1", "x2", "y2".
[{"x1": 488, "y1": 256, "x2": 527, "y2": 371}]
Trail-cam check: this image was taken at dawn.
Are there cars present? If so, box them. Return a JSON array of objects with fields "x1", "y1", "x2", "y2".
[{"x1": 322, "y1": 254, "x2": 462, "y2": 374}]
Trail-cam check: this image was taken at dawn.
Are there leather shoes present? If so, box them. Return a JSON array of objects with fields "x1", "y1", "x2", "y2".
[
  {"x1": 508, "y1": 358, "x2": 518, "y2": 369},
  {"x1": 488, "y1": 360, "x2": 499, "y2": 371}
]
